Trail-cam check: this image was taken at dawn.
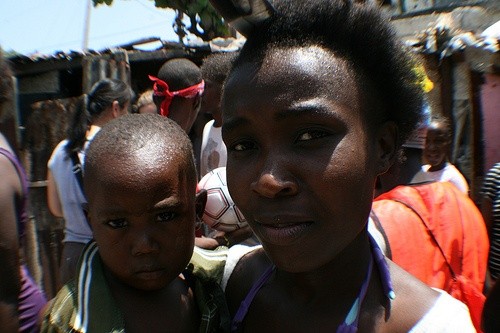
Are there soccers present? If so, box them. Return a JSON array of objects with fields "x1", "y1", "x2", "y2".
[{"x1": 196, "y1": 167, "x2": 251, "y2": 233}]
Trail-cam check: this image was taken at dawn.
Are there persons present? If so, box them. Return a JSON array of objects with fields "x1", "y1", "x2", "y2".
[
  {"x1": 0, "y1": 51, "x2": 500, "y2": 333},
  {"x1": 210, "y1": 0, "x2": 477, "y2": 333}
]
